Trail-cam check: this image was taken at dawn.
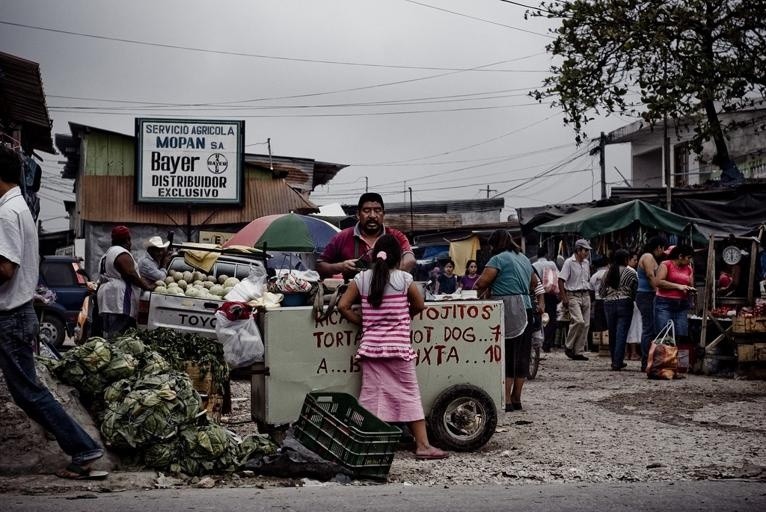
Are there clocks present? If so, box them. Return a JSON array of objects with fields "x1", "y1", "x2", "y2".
[{"x1": 723, "y1": 245, "x2": 742, "y2": 265}]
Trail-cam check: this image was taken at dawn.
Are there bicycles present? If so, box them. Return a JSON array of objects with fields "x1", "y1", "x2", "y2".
[{"x1": 525, "y1": 298, "x2": 550, "y2": 381}]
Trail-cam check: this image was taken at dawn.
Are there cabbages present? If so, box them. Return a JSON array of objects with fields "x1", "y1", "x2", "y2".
[{"x1": 49, "y1": 336, "x2": 279, "y2": 477}]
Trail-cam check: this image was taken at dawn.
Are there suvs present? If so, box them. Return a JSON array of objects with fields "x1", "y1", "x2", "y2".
[{"x1": 39, "y1": 252, "x2": 94, "y2": 350}]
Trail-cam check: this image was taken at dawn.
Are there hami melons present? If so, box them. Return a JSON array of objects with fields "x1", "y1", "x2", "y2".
[{"x1": 155, "y1": 270, "x2": 240, "y2": 301}]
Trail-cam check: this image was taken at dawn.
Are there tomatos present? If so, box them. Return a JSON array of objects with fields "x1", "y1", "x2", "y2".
[{"x1": 712, "y1": 305, "x2": 732, "y2": 313}]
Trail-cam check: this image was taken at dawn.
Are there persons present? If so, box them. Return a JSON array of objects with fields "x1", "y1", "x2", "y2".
[
  {"x1": 315, "y1": 192, "x2": 417, "y2": 285},
  {"x1": 91, "y1": 223, "x2": 158, "y2": 338},
  {"x1": 0, "y1": 145, "x2": 108, "y2": 481},
  {"x1": 137, "y1": 235, "x2": 171, "y2": 283},
  {"x1": 337, "y1": 234, "x2": 449, "y2": 460}
]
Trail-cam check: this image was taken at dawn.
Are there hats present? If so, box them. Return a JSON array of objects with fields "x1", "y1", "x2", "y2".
[
  {"x1": 142, "y1": 235, "x2": 171, "y2": 250},
  {"x1": 110, "y1": 225, "x2": 131, "y2": 245},
  {"x1": 661, "y1": 244, "x2": 677, "y2": 257},
  {"x1": 573, "y1": 238, "x2": 594, "y2": 250}
]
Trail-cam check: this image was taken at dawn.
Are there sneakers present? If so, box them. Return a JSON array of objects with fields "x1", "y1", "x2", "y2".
[
  {"x1": 610, "y1": 362, "x2": 627, "y2": 371},
  {"x1": 565, "y1": 349, "x2": 573, "y2": 359},
  {"x1": 572, "y1": 355, "x2": 589, "y2": 361},
  {"x1": 513, "y1": 401, "x2": 523, "y2": 410},
  {"x1": 505, "y1": 403, "x2": 515, "y2": 412}
]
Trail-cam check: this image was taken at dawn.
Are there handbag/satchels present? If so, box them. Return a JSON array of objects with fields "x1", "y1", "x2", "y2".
[
  {"x1": 646, "y1": 318, "x2": 680, "y2": 381},
  {"x1": 541, "y1": 266, "x2": 559, "y2": 294},
  {"x1": 307, "y1": 279, "x2": 349, "y2": 323}
]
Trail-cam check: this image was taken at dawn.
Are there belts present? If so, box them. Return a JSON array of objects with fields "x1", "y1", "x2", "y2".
[{"x1": 568, "y1": 288, "x2": 587, "y2": 294}]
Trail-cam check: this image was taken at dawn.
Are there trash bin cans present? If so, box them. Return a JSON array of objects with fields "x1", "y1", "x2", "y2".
[{"x1": 688, "y1": 317, "x2": 734, "y2": 375}]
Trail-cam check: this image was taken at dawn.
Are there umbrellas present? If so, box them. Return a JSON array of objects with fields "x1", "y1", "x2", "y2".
[{"x1": 220, "y1": 210, "x2": 342, "y2": 274}]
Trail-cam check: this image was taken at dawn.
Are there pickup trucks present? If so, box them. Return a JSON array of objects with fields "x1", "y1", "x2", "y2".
[{"x1": 143, "y1": 238, "x2": 269, "y2": 362}]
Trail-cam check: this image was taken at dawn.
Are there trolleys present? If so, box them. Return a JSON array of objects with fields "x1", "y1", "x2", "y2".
[{"x1": 234, "y1": 296, "x2": 512, "y2": 453}]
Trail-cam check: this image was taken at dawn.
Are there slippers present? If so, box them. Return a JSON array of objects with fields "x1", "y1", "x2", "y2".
[
  {"x1": 414, "y1": 447, "x2": 450, "y2": 459},
  {"x1": 53, "y1": 462, "x2": 111, "y2": 481}
]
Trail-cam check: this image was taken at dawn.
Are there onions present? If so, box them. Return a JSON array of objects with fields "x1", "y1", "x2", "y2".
[{"x1": 753, "y1": 304, "x2": 764, "y2": 316}]
